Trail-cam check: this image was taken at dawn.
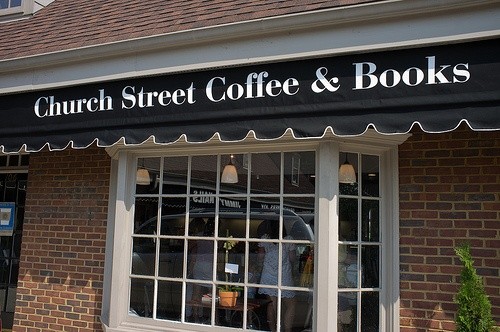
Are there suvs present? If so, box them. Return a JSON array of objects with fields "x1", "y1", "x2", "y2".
[{"x1": 130, "y1": 207, "x2": 315, "y2": 329}]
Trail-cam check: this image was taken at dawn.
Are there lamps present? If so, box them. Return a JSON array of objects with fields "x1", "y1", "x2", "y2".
[
  {"x1": 136, "y1": 159, "x2": 150, "y2": 185},
  {"x1": 339, "y1": 152, "x2": 357, "y2": 184},
  {"x1": 221, "y1": 155, "x2": 238, "y2": 183}
]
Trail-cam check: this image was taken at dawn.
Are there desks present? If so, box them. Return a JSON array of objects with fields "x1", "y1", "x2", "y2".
[{"x1": 186, "y1": 295, "x2": 273, "y2": 328}]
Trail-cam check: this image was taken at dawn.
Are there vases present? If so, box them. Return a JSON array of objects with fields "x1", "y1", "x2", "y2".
[{"x1": 218, "y1": 290, "x2": 239, "y2": 306}]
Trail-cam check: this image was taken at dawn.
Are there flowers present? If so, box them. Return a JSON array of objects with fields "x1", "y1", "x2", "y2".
[{"x1": 217, "y1": 229, "x2": 244, "y2": 292}]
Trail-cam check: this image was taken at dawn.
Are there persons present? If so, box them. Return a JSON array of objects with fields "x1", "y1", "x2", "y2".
[
  {"x1": 168, "y1": 218, "x2": 224, "y2": 327},
  {"x1": 338, "y1": 217, "x2": 366, "y2": 332},
  {"x1": 256, "y1": 221, "x2": 298, "y2": 332}
]
